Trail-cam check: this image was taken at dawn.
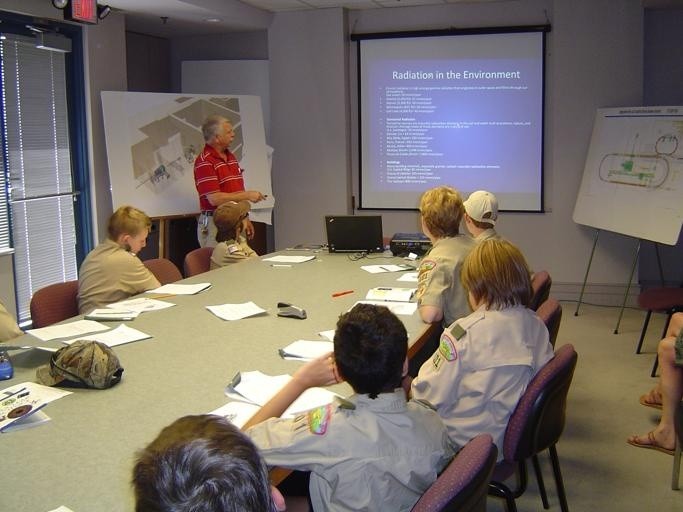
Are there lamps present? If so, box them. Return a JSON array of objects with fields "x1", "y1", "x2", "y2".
[{"x1": 50, "y1": 0, "x2": 111, "y2": 26}]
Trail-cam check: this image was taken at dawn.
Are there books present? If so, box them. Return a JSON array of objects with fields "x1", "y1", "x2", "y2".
[{"x1": 84, "y1": 308, "x2": 144, "y2": 321}]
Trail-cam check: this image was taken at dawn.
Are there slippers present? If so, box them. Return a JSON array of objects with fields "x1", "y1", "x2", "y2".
[
  {"x1": 627, "y1": 431, "x2": 674, "y2": 455},
  {"x1": 639, "y1": 392, "x2": 663, "y2": 409}
]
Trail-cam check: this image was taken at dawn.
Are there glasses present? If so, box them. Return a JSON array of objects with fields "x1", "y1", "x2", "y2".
[{"x1": 49, "y1": 347, "x2": 87, "y2": 386}]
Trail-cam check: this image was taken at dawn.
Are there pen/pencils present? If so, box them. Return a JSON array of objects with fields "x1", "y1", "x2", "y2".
[
  {"x1": 332, "y1": 290, "x2": 354, "y2": 297},
  {"x1": 145, "y1": 294, "x2": 176, "y2": 300},
  {"x1": 269, "y1": 264, "x2": 292, "y2": 267}
]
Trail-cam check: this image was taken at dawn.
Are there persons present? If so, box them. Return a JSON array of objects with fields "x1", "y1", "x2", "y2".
[
  {"x1": 417, "y1": 186, "x2": 479, "y2": 331},
  {"x1": 626, "y1": 312, "x2": 683, "y2": 455},
  {"x1": 131, "y1": 413, "x2": 287, "y2": 512},
  {"x1": 209, "y1": 200, "x2": 260, "y2": 272},
  {"x1": 193, "y1": 114, "x2": 266, "y2": 249},
  {"x1": 76, "y1": 206, "x2": 162, "y2": 316},
  {"x1": 463, "y1": 190, "x2": 504, "y2": 244},
  {"x1": 240, "y1": 303, "x2": 464, "y2": 511},
  {"x1": 408, "y1": 239, "x2": 555, "y2": 463}
]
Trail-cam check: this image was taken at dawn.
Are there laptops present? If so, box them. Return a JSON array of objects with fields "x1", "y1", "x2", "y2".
[{"x1": 325, "y1": 216, "x2": 385, "y2": 253}]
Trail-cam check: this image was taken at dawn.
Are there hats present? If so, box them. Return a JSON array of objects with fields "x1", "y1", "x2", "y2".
[
  {"x1": 36, "y1": 340, "x2": 123, "y2": 390},
  {"x1": 213, "y1": 200, "x2": 250, "y2": 232},
  {"x1": 462, "y1": 191, "x2": 498, "y2": 227}
]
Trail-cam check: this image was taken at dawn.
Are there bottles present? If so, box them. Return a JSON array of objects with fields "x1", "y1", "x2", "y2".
[{"x1": 0, "y1": 343, "x2": 13, "y2": 380}]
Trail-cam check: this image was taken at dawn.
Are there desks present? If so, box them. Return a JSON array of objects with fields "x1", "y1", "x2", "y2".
[{"x1": 0, "y1": 243, "x2": 445, "y2": 511}]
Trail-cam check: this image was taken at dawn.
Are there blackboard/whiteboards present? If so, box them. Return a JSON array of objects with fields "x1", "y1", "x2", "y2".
[{"x1": 100, "y1": 91, "x2": 275, "y2": 221}]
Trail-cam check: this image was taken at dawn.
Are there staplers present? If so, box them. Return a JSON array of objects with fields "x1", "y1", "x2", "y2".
[{"x1": 277, "y1": 303, "x2": 307, "y2": 319}]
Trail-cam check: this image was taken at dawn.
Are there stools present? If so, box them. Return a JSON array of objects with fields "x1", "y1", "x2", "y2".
[{"x1": 634, "y1": 279, "x2": 682, "y2": 381}]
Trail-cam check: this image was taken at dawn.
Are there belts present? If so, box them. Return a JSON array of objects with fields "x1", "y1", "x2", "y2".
[{"x1": 201, "y1": 211, "x2": 213, "y2": 216}]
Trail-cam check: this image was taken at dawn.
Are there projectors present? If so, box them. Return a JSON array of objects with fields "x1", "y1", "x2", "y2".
[{"x1": 389, "y1": 233, "x2": 434, "y2": 257}]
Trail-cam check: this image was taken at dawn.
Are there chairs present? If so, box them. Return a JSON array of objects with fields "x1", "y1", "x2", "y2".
[
  {"x1": 26, "y1": 276, "x2": 79, "y2": 328},
  {"x1": 523, "y1": 269, "x2": 553, "y2": 314},
  {"x1": 532, "y1": 296, "x2": 562, "y2": 354},
  {"x1": 403, "y1": 428, "x2": 498, "y2": 511},
  {"x1": 139, "y1": 254, "x2": 184, "y2": 287},
  {"x1": 483, "y1": 339, "x2": 582, "y2": 512},
  {"x1": 181, "y1": 245, "x2": 215, "y2": 283}
]
